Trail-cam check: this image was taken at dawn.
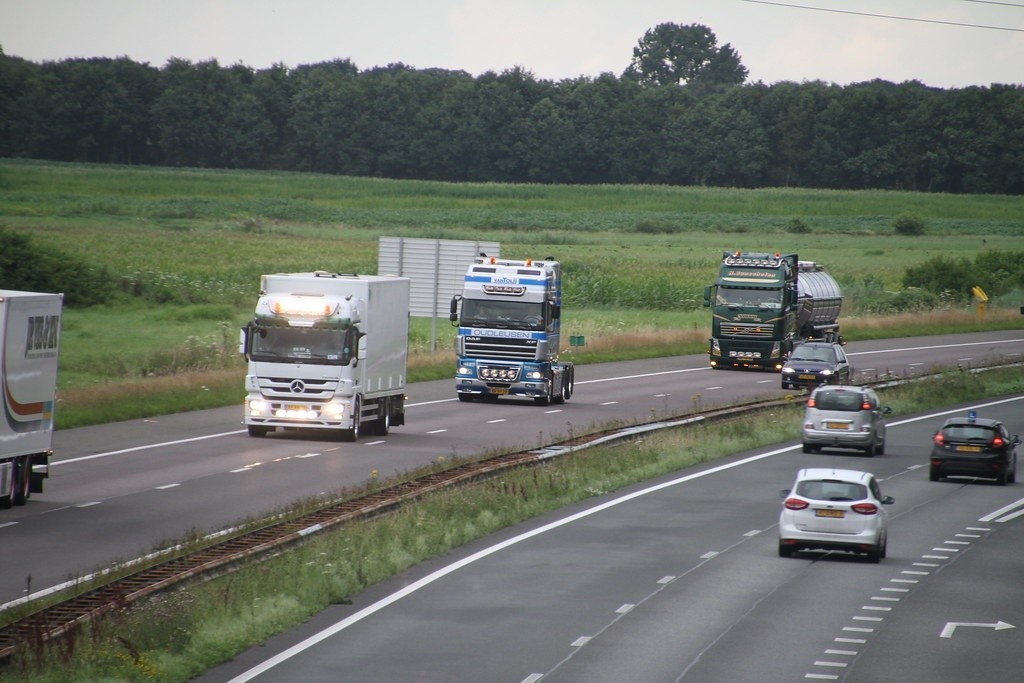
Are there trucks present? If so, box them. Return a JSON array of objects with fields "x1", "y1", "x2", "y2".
[
  {"x1": 702, "y1": 250, "x2": 847, "y2": 372},
  {"x1": 0, "y1": 289, "x2": 64, "y2": 509},
  {"x1": 238, "y1": 270, "x2": 411, "y2": 441}
]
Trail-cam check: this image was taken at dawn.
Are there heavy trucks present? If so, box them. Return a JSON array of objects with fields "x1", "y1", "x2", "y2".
[{"x1": 449, "y1": 253, "x2": 574, "y2": 406}]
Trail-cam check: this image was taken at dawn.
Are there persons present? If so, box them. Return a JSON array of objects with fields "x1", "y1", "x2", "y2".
[
  {"x1": 264, "y1": 329, "x2": 284, "y2": 354},
  {"x1": 325, "y1": 335, "x2": 353, "y2": 359},
  {"x1": 520, "y1": 306, "x2": 544, "y2": 330},
  {"x1": 472, "y1": 304, "x2": 488, "y2": 327}
]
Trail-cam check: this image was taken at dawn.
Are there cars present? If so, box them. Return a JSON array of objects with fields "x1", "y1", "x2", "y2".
[
  {"x1": 778, "y1": 468, "x2": 896, "y2": 563},
  {"x1": 928, "y1": 417, "x2": 1024, "y2": 485}
]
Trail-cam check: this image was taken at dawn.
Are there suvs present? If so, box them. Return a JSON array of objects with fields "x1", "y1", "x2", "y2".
[
  {"x1": 781, "y1": 340, "x2": 852, "y2": 389},
  {"x1": 801, "y1": 383, "x2": 892, "y2": 458}
]
